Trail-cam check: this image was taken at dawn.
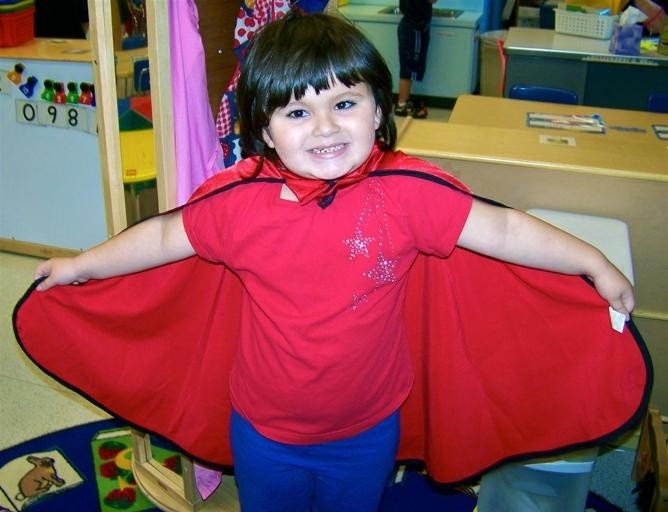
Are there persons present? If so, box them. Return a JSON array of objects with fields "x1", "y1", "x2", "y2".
[
  {"x1": 623, "y1": 0, "x2": 668, "y2": 37},
  {"x1": 34, "y1": 12, "x2": 634, "y2": 511},
  {"x1": 394, "y1": 0, "x2": 438, "y2": 120}
]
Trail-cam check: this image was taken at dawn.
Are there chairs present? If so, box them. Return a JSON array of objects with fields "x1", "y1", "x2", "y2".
[{"x1": 114, "y1": 46, "x2": 158, "y2": 224}]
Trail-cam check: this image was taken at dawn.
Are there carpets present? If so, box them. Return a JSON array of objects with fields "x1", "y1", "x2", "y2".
[{"x1": 0, "y1": 417, "x2": 627, "y2": 512}]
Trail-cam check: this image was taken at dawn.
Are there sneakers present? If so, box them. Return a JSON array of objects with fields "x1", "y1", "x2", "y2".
[{"x1": 394, "y1": 100, "x2": 429, "y2": 119}]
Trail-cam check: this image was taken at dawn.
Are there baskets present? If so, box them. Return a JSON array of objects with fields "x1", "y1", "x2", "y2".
[{"x1": 552, "y1": 6, "x2": 620, "y2": 41}]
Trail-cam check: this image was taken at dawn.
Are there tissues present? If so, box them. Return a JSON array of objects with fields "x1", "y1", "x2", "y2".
[{"x1": 609, "y1": 5, "x2": 650, "y2": 57}]
